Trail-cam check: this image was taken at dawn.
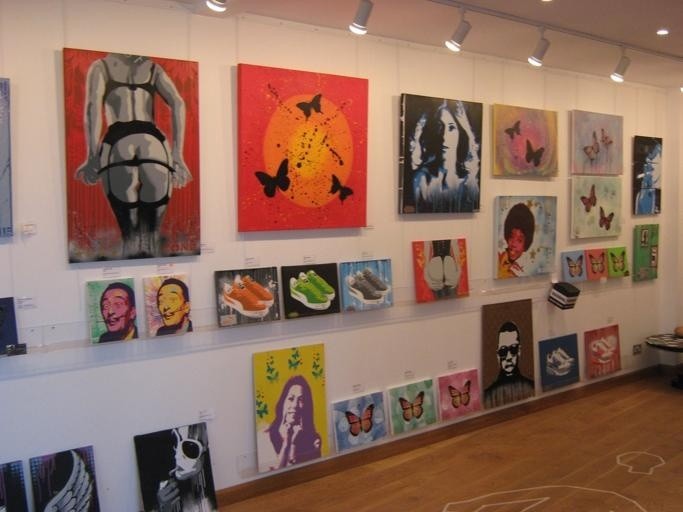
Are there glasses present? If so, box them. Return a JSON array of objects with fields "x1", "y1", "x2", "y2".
[{"x1": 497, "y1": 343, "x2": 520, "y2": 356}]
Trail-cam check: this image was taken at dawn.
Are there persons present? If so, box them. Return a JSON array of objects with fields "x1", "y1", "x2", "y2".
[
  {"x1": 254, "y1": 373, "x2": 322, "y2": 475},
  {"x1": 406, "y1": 97, "x2": 481, "y2": 213},
  {"x1": 420, "y1": 239, "x2": 464, "y2": 300},
  {"x1": 478, "y1": 319, "x2": 535, "y2": 408},
  {"x1": 72, "y1": 51, "x2": 196, "y2": 260},
  {"x1": 154, "y1": 278, "x2": 192, "y2": 334},
  {"x1": 153, "y1": 421, "x2": 216, "y2": 510},
  {"x1": 496, "y1": 203, "x2": 535, "y2": 277},
  {"x1": 92, "y1": 280, "x2": 141, "y2": 342}
]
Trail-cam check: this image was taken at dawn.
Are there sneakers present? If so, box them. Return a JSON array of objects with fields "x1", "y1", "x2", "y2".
[
  {"x1": 234, "y1": 274, "x2": 274, "y2": 308},
  {"x1": 363, "y1": 267, "x2": 390, "y2": 293},
  {"x1": 289, "y1": 277, "x2": 332, "y2": 311},
  {"x1": 553, "y1": 348, "x2": 574, "y2": 367},
  {"x1": 222, "y1": 283, "x2": 270, "y2": 318},
  {"x1": 546, "y1": 352, "x2": 569, "y2": 376},
  {"x1": 298, "y1": 271, "x2": 336, "y2": 300},
  {"x1": 347, "y1": 272, "x2": 386, "y2": 305}
]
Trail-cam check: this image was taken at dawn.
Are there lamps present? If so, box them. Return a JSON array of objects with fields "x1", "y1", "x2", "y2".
[{"x1": 206, "y1": 0, "x2": 631, "y2": 85}]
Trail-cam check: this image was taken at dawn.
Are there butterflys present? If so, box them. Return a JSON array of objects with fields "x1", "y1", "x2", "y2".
[
  {"x1": 448, "y1": 381, "x2": 471, "y2": 408},
  {"x1": 589, "y1": 252, "x2": 606, "y2": 274},
  {"x1": 610, "y1": 251, "x2": 626, "y2": 272},
  {"x1": 525, "y1": 138, "x2": 545, "y2": 167},
  {"x1": 503, "y1": 120, "x2": 521, "y2": 140},
  {"x1": 601, "y1": 129, "x2": 613, "y2": 152},
  {"x1": 566, "y1": 255, "x2": 584, "y2": 277},
  {"x1": 297, "y1": 94, "x2": 324, "y2": 122},
  {"x1": 345, "y1": 404, "x2": 376, "y2": 436},
  {"x1": 599, "y1": 207, "x2": 614, "y2": 230},
  {"x1": 582, "y1": 129, "x2": 600, "y2": 163},
  {"x1": 399, "y1": 392, "x2": 427, "y2": 421},
  {"x1": 579, "y1": 184, "x2": 598, "y2": 212},
  {"x1": 329, "y1": 174, "x2": 354, "y2": 203},
  {"x1": 255, "y1": 158, "x2": 290, "y2": 198}
]
